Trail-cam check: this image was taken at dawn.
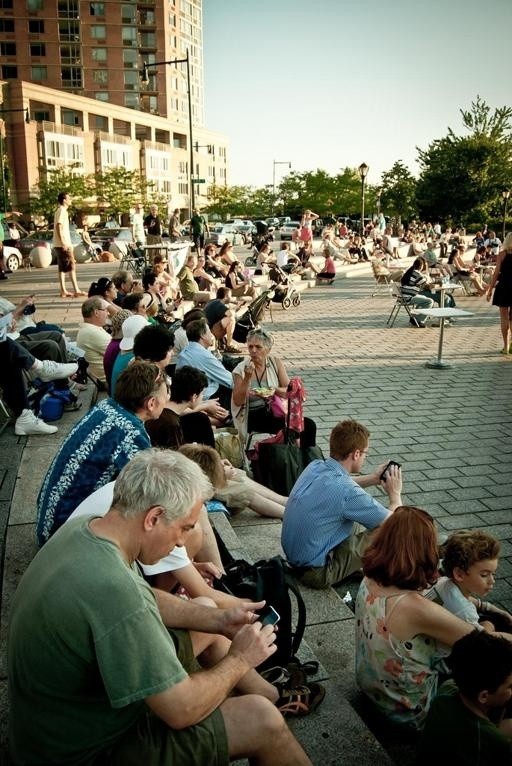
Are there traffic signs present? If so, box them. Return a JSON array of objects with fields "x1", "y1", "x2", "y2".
[{"x1": 191, "y1": 178, "x2": 206, "y2": 184}]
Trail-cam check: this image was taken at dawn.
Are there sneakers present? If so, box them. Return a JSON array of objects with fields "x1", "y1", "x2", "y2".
[
  {"x1": 15, "y1": 409, "x2": 58, "y2": 436},
  {"x1": 258, "y1": 666, "x2": 325, "y2": 721},
  {"x1": 61, "y1": 291, "x2": 87, "y2": 298},
  {"x1": 39, "y1": 359, "x2": 80, "y2": 383}
]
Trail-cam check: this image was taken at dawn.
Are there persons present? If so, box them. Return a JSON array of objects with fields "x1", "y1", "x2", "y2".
[
  {"x1": 62, "y1": 443, "x2": 252, "y2": 611},
  {"x1": 486, "y1": 231, "x2": 507, "y2": 354},
  {"x1": 8, "y1": 449, "x2": 315, "y2": 765},
  {"x1": 419, "y1": 530, "x2": 512, "y2": 651},
  {"x1": 428, "y1": 627, "x2": 511, "y2": 766},
  {"x1": 355, "y1": 506, "x2": 510, "y2": 735},
  {"x1": 280, "y1": 421, "x2": 402, "y2": 589},
  {"x1": 1, "y1": 202, "x2": 503, "y2": 543},
  {"x1": 54, "y1": 194, "x2": 87, "y2": 297}
]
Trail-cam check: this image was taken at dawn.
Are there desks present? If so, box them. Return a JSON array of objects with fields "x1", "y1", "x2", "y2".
[
  {"x1": 411, "y1": 308, "x2": 475, "y2": 369},
  {"x1": 143, "y1": 243, "x2": 190, "y2": 277}
]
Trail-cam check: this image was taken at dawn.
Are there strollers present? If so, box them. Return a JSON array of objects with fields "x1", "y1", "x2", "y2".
[
  {"x1": 260, "y1": 261, "x2": 301, "y2": 311},
  {"x1": 232, "y1": 283, "x2": 284, "y2": 343},
  {"x1": 244, "y1": 247, "x2": 274, "y2": 266}
]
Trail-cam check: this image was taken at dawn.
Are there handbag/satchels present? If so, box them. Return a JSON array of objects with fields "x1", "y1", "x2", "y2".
[
  {"x1": 270, "y1": 396, "x2": 284, "y2": 417},
  {"x1": 250, "y1": 442, "x2": 326, "y2": 497},
  {"x1": 213, "y1": 555, "x2": 292, "y2": 671}
]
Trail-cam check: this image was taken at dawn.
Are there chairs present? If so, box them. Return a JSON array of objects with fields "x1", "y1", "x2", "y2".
[
  {"x1": 113, "y1": 240, "x2": 142, "y2": 278},
  {"x1": 370, "y1": 258, "x2": 496, "y2": 328}
]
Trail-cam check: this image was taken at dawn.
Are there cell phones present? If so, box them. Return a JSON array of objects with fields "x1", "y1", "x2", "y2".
[
  {"x1": 379, "y1": 461, "x2": 402, "y2": 482},
  {"x1": 251, "y1": 605, "x2": 281, "y2": 631}
]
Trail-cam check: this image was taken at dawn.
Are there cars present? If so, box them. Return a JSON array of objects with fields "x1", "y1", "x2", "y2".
[
  {"x1": 164, "y1": 215, "x2": 394, "y2": 248},
  {"x1": 0, "y1": 215, "x2": 164, "y2": 274}
]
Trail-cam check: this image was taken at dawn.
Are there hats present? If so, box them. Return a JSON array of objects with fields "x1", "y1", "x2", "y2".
[
  {"x1": 111, "y1": 309, "x2": 148, "y2": 351},
  {"x1": 203, "y1": 299, "x2": 229, "y2": 325}
]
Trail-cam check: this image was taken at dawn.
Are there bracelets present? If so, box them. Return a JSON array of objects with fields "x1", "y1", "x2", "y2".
[{"x1": 477, "y1": 597, "x2": 489, "y2": 612}]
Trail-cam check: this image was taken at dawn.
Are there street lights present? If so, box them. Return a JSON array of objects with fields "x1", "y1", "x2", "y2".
[
  {"x1": 499, "y1": 184, "x2": 511, "y2": 242},
  {"x1": 358, "y1": 160, "x2": 370, "y2": 236},
  {"x1": 272, "y1": 159, "x2": 292, "y2": 196},
  {"x1": 0, "y1": 106, "x2": 30, "y2": 213},
  {"x1": 138, "y1": 47, "x2": 196, "y2": 213},
  {"x1": 189, "y1": 143, "x2": 213, "y2": 212}
]
